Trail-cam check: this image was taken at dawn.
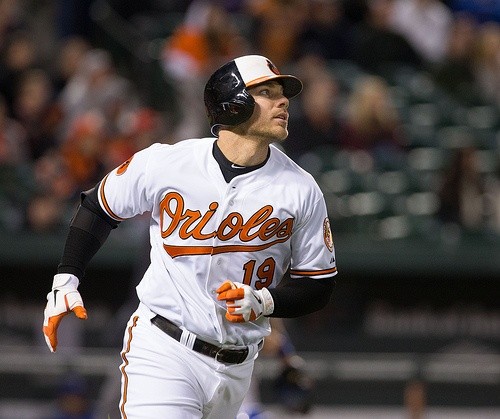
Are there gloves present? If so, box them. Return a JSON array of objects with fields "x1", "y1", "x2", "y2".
[
  {"x1": 215, "y1": 281, "x2": 274, "y2": 323},
  {"x1": 42, "y1": 272, "x2": 87, "y2": 353}
]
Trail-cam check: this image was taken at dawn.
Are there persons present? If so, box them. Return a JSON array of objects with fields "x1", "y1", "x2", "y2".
[{"x1": 42, "y1": 55, "x2": 338, "y2": 419}]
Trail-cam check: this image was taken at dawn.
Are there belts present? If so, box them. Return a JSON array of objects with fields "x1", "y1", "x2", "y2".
[{"x1": 150, "y1": 315, "x2": 263, "y2": 365}]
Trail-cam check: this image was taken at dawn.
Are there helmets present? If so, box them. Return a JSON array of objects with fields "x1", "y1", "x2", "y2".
[{"x1": 203, "y1": 55, "x2": 304, "y2": 138}]
